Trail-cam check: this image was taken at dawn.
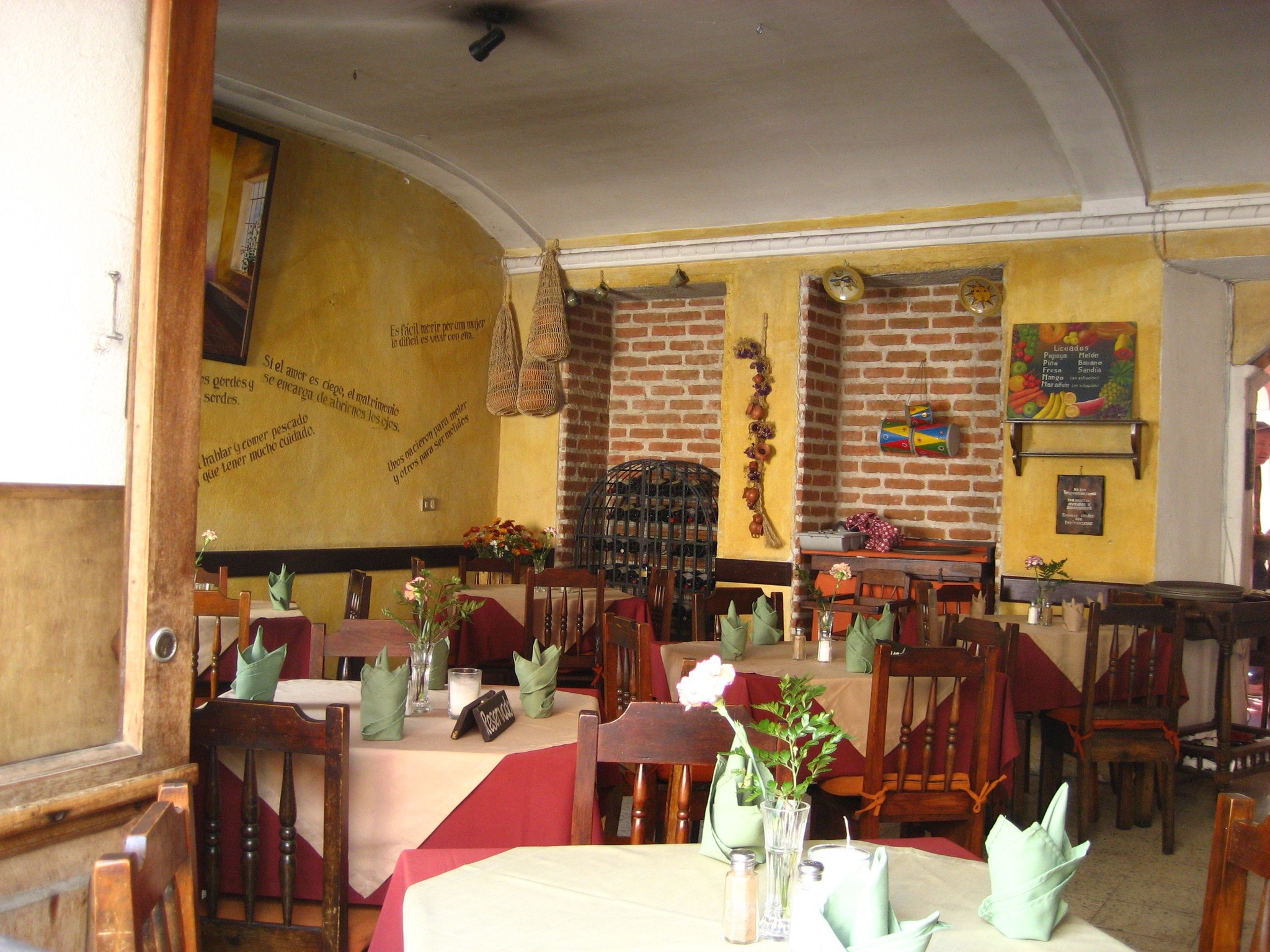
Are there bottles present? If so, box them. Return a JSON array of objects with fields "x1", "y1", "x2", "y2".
[
  {"x1": 579, "y1": 563, "x2": 652, "y2": 584},
  {"x1": 792, "y1": 627, "x2": 806, "y2": 660},
  {"x1": 590, "y1": 535, "x2": 654, "y2": 553},
  {"x1": 559, "y1": 587, "x2": 573, "y2": 593},
  {"x1": 674, "y1": 570, "x2": 715, "y2": 591},
  {"x1": 672, "y1": 479, "x2": 705, "y2": 497},
  {"x1": 622, "y1": 505, "x2": 654, "y2": 520},
  {"x1": 670, "y1": 508, "x2": 695, "y2": 523},
  {"x1": 645, "y1": 504, "x2": 669, "y2": 519},
  {"x1": 656, "y1": 478, "x2": 684, "y2": 496},
  {"x1": 669, "y1": 539, "x2": 717, "y2": 557},
  {"x1": 1042, "y1": 602, "x2": 1052, "y2": 626},
  {"x1": 645, "y1": 478, "x2": 675, "y2": 494},
  {"x1": 607, "y1": 479, "x2": 656, "y2": 494},
  {"x1": 657, "y1": 505, "x2": 683, "y2": 522},
  {"x1": 688, "y1": 507, "x2": 717, "y2": 523},
  {"x1": 787, "y1": 860, "x2": 824, "y2": 943},
  {"x1": 817, "y1": 630, "x2": 832, "y2": 663},
  {"x1": 1027, "y1": 601, "x2": 1039, "y2": 625},
  {"x1": 722, "y1": 850, "x2": 759, "y2": 945},
  {"x1": 606, "y1": 503, "x2": 639, "y2": 519},
  {"x1": 671, "y1": 602, "x2": 692, "y2": 621}
]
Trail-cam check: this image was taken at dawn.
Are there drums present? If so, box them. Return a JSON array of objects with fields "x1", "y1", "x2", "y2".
[
  {"x1": 912, "y1": 422, "x2": 961, "y2": 458},
  {"x1": 905, "y1": 402, "x2": 933, "y2": 427},
  {"x1": 879, "y1": 420, "x2": 914, "y2": 454}
]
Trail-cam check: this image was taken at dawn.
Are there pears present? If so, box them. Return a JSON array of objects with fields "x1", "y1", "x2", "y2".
[{"x1": 1114, "y1": 333, "x2": 1134, "y2": 350}]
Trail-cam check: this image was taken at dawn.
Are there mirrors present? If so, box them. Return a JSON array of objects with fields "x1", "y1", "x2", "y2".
[{"x1": 207, "y1": 107, "x2": 283, "y2": 368}]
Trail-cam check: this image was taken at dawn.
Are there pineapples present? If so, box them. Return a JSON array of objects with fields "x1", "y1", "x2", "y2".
[{"x1": 1098, "y1": 360, "x2": 1134, "y2": 411}]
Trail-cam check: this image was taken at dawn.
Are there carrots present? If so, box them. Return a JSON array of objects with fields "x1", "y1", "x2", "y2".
[{"x1": 1009, "y1": 386, "x2": 1043, "y2": 415}]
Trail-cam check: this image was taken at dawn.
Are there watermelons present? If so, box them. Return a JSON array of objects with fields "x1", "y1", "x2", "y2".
[{"x1": 1074, "y1": 397, "x2": 1105, "y2": 416}]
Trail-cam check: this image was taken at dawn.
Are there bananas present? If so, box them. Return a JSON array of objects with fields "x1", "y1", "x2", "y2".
[{"x1": 1032, "y1": 391, "x2": 1066, "y2": 419}]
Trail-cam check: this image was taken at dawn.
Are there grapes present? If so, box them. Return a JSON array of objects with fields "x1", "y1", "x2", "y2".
[{"x1": 1094, "y1": 403, "x2": 1128, "y2": 419}]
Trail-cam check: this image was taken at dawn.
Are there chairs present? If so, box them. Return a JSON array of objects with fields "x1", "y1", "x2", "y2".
[
  {"x1": 598, "y1": 607, "x2": 657, "y2": 835},
  {"x1": 566, "y1": 691, "x2": 801, "y2": 846},
  {"x1": 813, "y1": 640, "x2": 1009, "y2": 845},
  {"x1": 85, "y1": 776, "x2": 206, "y2": 952},
  {"x1": 692, "y1": 585, "x2": 786, "y2": 641},
  {"x1": 191, "y1": 694, "x2": 386, "y2": 952},
  {"x1": 193, "y1": 586, "x2": 253, "y2": 706},
  {"x1": 193, "y1": 564, "x2": 229, "y2": 597},
  {"x1": 330, "y1": 564, "x2": 375, "y2": 680},
  {"x1": 1042, "y1": 595, "x2": 1194, "y2": 856},
  {"x1": 307, "y1": 615, "x2": 450, "y2": 681},
  {"x1": 516, "y1": 564, "x2": 608, "y2": 692}
]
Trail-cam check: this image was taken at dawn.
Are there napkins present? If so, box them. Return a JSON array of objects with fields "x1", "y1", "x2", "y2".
[
  {"x1": 356, "y1": 641, "x2": 411, "y2": 741},
  {"x1": 966, "y1": 588, "x2": 988, "y2": 617},
  {"x1": 509, "y1": 636, "x2": 565, "y2": 720},
  {"x1": 716, "y1": 596, "x2": 750, "y2": 660},
  {"x1": 750, "y1": 592, "x2": 783, "y2": 645},
  {"x1": 813, "y1": 844, "x2": 954, "y2": 952},
  {"x1": 267, "y1": 562, "x2": 297, "y2": 611},
  {"x1": 694, "y1": 714, "x2": 773, "y2": 867},
  {"x1": 976, "y1": 768, "x2": 1089, "y2": 945},
  {"x1": 869, "y1": 599, "x2": 896, "y2": 641},
  {"x1": 229, "y1": 622, "x2": 289, "y2": 704},
  {"x1": 841, "y1": 611, "x2": 877, "y2": 674},
  {"x1": 428, "y1": 633, "x2": 453, "y2": 690},
  {"x1": 1060, "y1": 596, "x2": 1087, "y2": 633}
]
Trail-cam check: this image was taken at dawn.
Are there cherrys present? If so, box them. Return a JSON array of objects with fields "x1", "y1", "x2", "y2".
[{"x1": 1023, "y1": 368, "x2": 1041, "y2": 388}]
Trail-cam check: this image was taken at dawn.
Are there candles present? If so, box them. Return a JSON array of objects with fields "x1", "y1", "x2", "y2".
[{"x1": 806, "y1": 815, "x2": 875, "y2": 888}]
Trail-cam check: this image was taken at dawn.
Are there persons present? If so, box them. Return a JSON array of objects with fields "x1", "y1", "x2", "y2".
[{"x1": 1254, "y1": 421, "x2": 1270, "y2": 591}]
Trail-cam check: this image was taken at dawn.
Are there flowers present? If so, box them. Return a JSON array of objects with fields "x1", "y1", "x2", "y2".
[
  {"x1": 460, "y1": 519, "x2": 556, "y2": 565},
  {"x1": 1022, "y1": 552, "x2": 1072, "y2": 601},
  {"x1": 676, "y1": 646, "x2": 859, "y2": 923},
  {"x1": 380, "y1": 567, "x2": 487, "y2": 700},
  {"x1": 789, "y1": 558, "x2": 851, "y2": 610}
]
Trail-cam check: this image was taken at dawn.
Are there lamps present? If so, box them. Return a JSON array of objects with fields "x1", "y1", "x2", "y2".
[{"x1": 463, "y1": 6, "x2": 512, "y2": 66}]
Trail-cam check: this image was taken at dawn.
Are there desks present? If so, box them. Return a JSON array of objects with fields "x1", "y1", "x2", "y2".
[{"x1": 192, "y1": 540, "x2": 1208, "y2": 952}]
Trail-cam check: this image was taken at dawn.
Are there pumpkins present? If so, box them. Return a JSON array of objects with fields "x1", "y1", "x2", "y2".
[
  {"x1": 487, "y1": 365, "x2": 519, "y2": 415},
  {"x1": 518, "y1": 365, "x2": 558, "y2": 417},
  {"x1": 531, "y1": 301, "x2": 570, "y2": 361}
]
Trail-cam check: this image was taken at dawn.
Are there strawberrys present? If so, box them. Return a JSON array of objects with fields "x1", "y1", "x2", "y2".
[{"x1": 1012, "y1": 342, "x2": 1034, "y2": 362}]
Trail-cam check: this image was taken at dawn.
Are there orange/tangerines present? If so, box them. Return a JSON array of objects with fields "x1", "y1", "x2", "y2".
[
  {"x1": 1064, "y1": 392, "x2": 1077, "y2": 405},
  {"x1": 1065, "y1": 405, "x2": 1080, "y2": 418}
]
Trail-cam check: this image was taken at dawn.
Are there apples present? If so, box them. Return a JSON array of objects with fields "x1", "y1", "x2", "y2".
[
  {"x1": 1036, "y1": 393, "x2": 1049, "y2": 407},
  {"x1": 1009, "y1": 375, "x2": 1025, "y2": 392},
  {"x1": 1063, "y1": 322, "x2": 1134, "y2": 360},
  {"x1": 1010, "y1": 361, "x2": 1027, "y2": 376},
  {"x1": 1023, "y1": 402, "x2": 1038, "y2": 417}
]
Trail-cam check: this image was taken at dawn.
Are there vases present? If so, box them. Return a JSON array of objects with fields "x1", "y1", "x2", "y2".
[
  {"x1": 403, "y1": 640, "x2": 435, "y2": 713},
  {"x1": 1034, "y1": 585, "x2": 1050, "y2": 622},
  {"x1": 815, "y1": 611, "x2": 834, "y2": 661},
  {"x1": 758, "y1": 799, "x2": 813, "y2": 941}
]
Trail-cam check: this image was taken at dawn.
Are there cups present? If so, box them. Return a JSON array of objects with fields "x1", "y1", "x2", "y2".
[
  {"x1": 195, "y1": 583, "x2": 214, "y2": 590},
  {"x1": 447, "y1": 668, "x2": 482, "y2": 720},
  {"x1": 808, "y1": 843, "x2": 873, "y2": 914},
  {"x1": 1062, "y1": 602, "x2": 1072, "y2": 622}
]
